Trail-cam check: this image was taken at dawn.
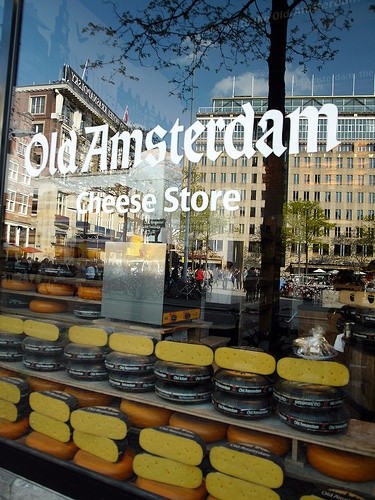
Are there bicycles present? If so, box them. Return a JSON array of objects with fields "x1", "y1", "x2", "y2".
[{"x1": 164, "y1": 273, "x2": 213, "y2": 300}]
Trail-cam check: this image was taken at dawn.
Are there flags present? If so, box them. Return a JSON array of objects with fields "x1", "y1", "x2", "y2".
[{"x1": 123, "y1": 107, "x2": 129, "y2": 124}]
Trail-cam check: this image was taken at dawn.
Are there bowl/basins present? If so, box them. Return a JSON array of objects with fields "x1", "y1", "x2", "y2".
[{"x1": 294, "y1": 347, "x2": 337, "y2": 360}]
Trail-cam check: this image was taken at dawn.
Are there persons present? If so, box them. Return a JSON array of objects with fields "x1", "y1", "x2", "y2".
[{"x1": 0, "y1": 243, "x2": 375, "y2": 322}]
